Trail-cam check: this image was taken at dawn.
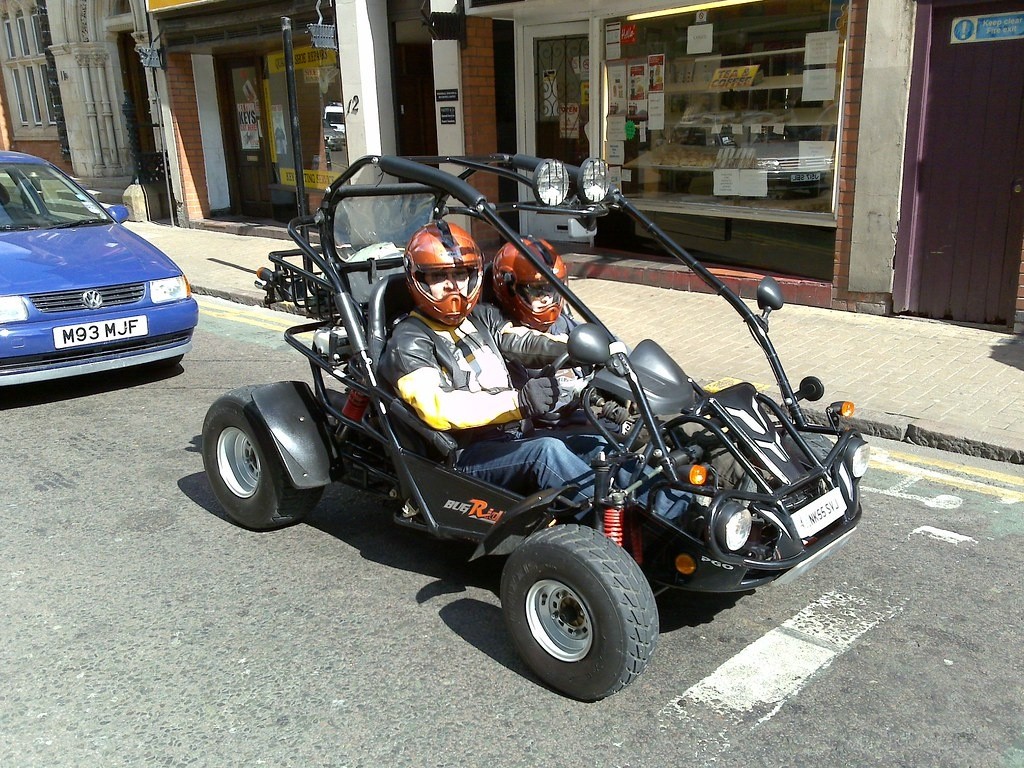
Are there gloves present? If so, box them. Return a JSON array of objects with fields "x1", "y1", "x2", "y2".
[{"x1": 518, "y1": 365, "x2": 559, "y2": 420}]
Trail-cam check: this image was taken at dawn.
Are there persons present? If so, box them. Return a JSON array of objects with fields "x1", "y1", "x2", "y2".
[
  {"x1": 490, "y1": 235, "x2": 778, "y2": 493},
  {"x1": 378, "y1": 220, "x2": 718, "y2": 527}
]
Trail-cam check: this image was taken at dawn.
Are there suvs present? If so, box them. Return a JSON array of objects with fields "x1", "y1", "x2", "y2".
[{"x1": 665, "y1": 108, "x2": 830, "y2": 198}]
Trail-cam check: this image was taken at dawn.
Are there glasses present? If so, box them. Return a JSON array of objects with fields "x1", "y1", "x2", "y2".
[
  {"x1": 523, "y1": 284, "x2": 556, "y2": 297},
  {"x1": 431, "y1": 270, "x2": 468, "y2": 282}
]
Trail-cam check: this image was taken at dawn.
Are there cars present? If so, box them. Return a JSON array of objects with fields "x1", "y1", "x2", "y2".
[{"x1": 0, "y1": 151, "x2": 199, "y2": 388}]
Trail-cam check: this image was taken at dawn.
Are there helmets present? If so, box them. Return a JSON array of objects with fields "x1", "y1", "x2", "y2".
[
  {"x1": 404, "y1": 219, "x2": 485, "y2": 325},
  {"x1": 493, "y1": 239, "x2": 568, "y2": 333}
]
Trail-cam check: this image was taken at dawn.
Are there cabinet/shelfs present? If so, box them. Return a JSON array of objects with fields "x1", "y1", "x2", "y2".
[{"x1": 620, "y1": 48, "x2": 833, "y2": 218}]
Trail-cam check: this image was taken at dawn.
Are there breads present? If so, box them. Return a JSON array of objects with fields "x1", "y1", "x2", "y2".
[{"x1": 646, "y1": 107, "x2": 791, "y2": 168}]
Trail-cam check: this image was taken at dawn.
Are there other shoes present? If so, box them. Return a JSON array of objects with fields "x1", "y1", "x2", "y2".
[
  {"x1": 730, "y1": 468, "x2": 764, "y2": 509},
  {"x1": 681, "y1": 493, "x2": 712, "y2": 534}
]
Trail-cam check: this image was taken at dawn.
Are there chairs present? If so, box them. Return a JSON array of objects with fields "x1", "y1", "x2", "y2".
[
  {"x1": 370, "y1": 270, "x2": 448, "y2": 462},
  {"x1": 0, "y1": 184, "x2": 41, "y2": 225}
]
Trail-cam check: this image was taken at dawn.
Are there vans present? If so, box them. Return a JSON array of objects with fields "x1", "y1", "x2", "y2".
[{"x1": 323, "y1": 104, "x2": 347, "y2": 152}]
